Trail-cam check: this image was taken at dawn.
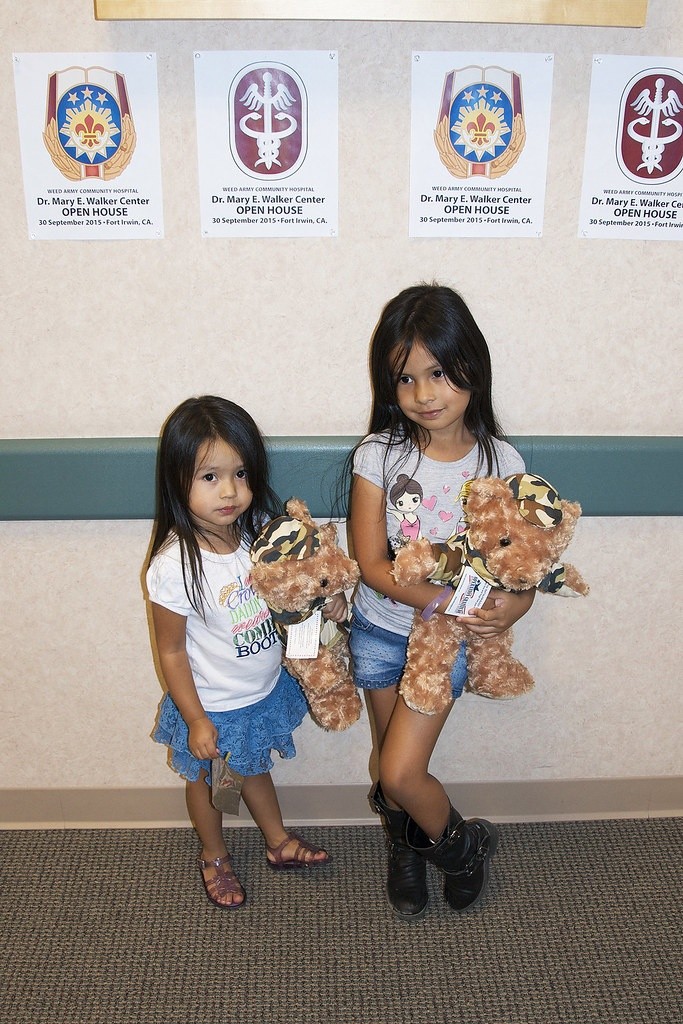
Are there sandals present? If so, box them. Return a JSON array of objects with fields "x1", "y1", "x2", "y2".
[
  {"x1": 265, "y1": 834, "x2": 333, "y2": 869},
  {"x1": 196, "y1": 853, "x2": 247, "y2": 910}
]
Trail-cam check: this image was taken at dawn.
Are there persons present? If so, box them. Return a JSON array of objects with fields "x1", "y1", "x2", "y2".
[
  {"x1": 147, "y1": 396, "x2": 352, "y2": 909},
  {"x1": 347, "y1": 286, "x2": 527, "y2": 918}
]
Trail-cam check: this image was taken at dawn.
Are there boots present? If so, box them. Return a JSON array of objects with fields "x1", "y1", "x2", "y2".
[
  {"x1": 406, "y1": 805, "x2": 498, "y2": 912},
  {"x1": 371, "y1": 780, "x2": 429, "y2": 920}
]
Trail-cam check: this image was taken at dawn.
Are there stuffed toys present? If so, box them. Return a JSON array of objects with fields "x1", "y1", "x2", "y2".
[
  {"x1": 390, "y1": 474, "x2": 590, "y2": 713},
  {"x1": 247, "y1": 493, "x2": 364, "y2": 731}
]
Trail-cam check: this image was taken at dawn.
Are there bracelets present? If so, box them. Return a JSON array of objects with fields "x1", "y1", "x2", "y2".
[{"x1": 421, "y1": 586, "x2": 452, "y2": 621}]
